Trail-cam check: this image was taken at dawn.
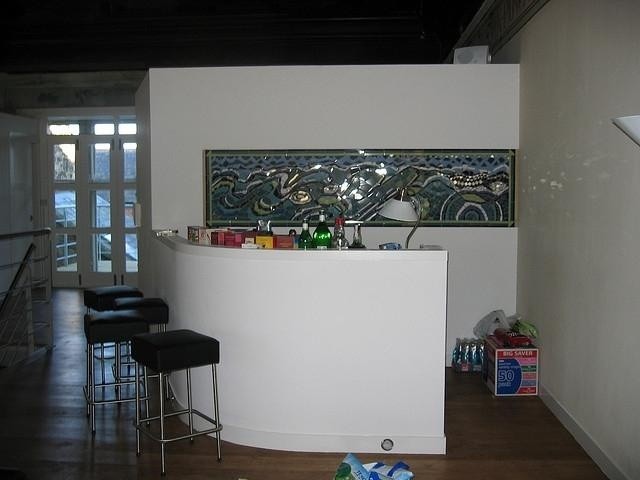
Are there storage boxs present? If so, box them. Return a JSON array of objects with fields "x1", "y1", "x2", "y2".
[{"x1": 482, "y1": 335, "x2": 539, "y2": 397}]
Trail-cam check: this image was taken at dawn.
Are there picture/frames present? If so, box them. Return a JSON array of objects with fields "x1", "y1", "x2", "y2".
[{"x1": 205, "y1": 149, "x2": 516, "y2": 227}]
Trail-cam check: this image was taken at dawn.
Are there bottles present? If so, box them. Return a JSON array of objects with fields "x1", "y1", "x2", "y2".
[
  {"x1": 452, "y1": 338, "x2": 485, "y2": 372},
  {"x1": 298, "y1": 209, "x2": 366, "y2": 250}
]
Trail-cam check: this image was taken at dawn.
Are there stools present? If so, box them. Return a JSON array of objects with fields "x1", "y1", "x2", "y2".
[
  {"x1": 131, "y1": 330, "x2": 223, "y2": 477},
  {"x1": 83, "y1": 286, "x2": 169, "y2": 436}
]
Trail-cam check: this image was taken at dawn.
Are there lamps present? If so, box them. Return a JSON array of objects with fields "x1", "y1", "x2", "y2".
[{"x1": 378, "y1": 187, "x2": 421, "y2": 249}]
